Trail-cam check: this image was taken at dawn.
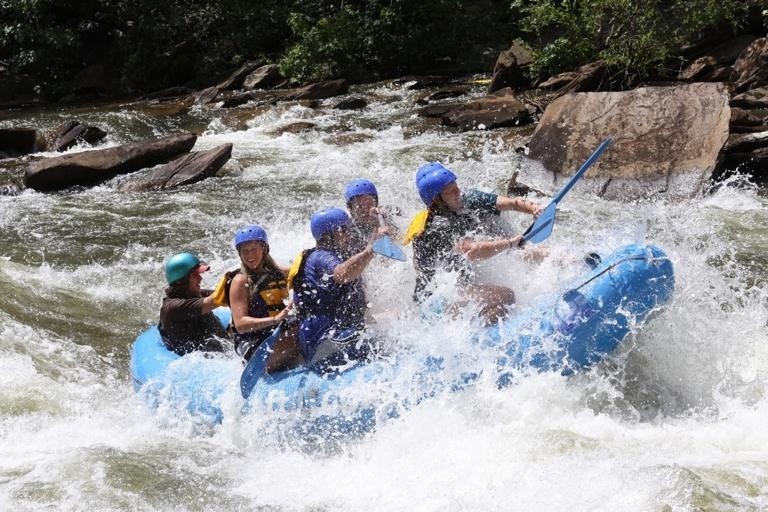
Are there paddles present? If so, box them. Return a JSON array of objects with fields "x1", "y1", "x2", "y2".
[
  {"x1": 239, "y1": 321, "x2": 284, "y2": 399},
  {"x1": 521, "y1": 201, "x2": 557, "y2": 245},
  {"x1": 372, "y1": 214, "x2": 408, "y2": 264}
]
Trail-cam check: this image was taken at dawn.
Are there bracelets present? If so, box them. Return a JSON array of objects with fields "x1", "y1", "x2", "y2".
[{"x1": 508, "y1": 239, "x2": 513, "y2": 248}]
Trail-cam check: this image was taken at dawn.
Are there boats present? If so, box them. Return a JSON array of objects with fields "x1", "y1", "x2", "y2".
[{"x1": 123, "y1": 241, "x2": 676, "y2": 454}]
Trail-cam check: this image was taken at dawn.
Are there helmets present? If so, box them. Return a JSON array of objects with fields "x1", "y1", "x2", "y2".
[
  {"x1": 344, "y1": 179, "x2": 378, "y2": 207},
  {"x1": 415, "y1": 162, "x2": 458, "y2": 207},
  {"x1": 164, "y1": 253, "x2": 201, "y2": 285},
  {"x1": 309, "y1": 207, "x2": 353, "y2": 241},
  {"x1": 233, "y1": 225, "x2": 268, "y2": 250}
]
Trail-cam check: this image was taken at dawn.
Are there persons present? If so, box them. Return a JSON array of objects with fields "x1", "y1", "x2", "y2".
[
  {"x1": 224, "y1": 225, "x2": 295, "y2": 363},
  {"x1": 412, "y1": 168, "x2": 524, "y2": 329},
  {"x1": 293, "y1": 208, "x2": 388, "y2": 381},
  {"x1": 345, "y1": 179, "x2": 405, "y2": 268},
  {"x1": 158, "y1": 252, "x2": 241, "y2": 356},
  {"x1": 416, "y1": 163, "x2": 549, "y2": 265}
]
